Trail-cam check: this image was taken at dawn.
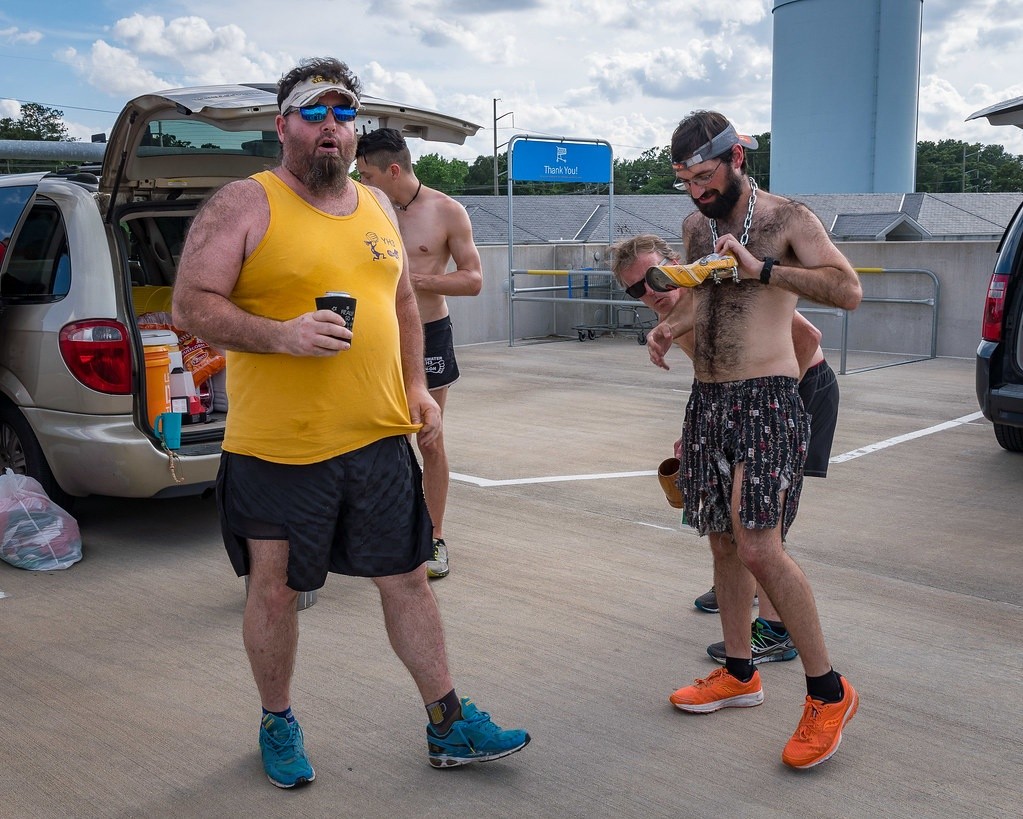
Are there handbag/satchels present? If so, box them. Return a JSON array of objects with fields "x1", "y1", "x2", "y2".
[{"x1": 0, "y1": 468, "x2": 82, "y2": 572}]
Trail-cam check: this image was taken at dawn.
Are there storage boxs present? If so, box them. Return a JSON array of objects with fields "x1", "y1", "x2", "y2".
[{"x1": 170, "y1": 350, "x2": 207, "y2": 425}]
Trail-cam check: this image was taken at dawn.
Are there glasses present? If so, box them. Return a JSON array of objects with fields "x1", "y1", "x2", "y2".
[
  {"x1": 626, "y1": 257, "x2": 669, "y2": 300},
  {"x1": 673, "y1": 158, "x2": 722, "y2": 191},
  {"x1": 390, "y1": 200, "x2": 406, "y2": 211},
  {"x1": 282, "y1": 101, "x2": 359, "y2": 123}
]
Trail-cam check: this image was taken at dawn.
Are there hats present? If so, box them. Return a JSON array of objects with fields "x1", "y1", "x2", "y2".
[
  {"x1": 280, "y1": 75, "x2": 360, "y2": 115},
  {"x1": 672, "y1": 122, "x2": 759, "y2": 170}
]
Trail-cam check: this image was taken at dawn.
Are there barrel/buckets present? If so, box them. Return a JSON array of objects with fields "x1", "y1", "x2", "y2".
[{"x1": 142, "y1": 345, "x2": 172, "y2": 432}]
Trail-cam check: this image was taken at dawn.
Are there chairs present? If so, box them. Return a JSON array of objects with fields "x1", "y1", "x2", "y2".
[{"x1": 120, "y1": 225, "x2": 148, "y2": 288}]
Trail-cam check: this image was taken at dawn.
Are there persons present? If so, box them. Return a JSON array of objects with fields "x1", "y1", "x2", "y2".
[
  {"x1": 648, "y1": 111, "x2": 863, "y2": 766},
  {"x1": 356, "y1": 128, "x2": 482, "y2": 578},
  {"x1": 172, "y1": 60, "x2": 532, "y2": 788},
  {"x1": 614, "y1": 235, "x2": 840, "y2": 663}
]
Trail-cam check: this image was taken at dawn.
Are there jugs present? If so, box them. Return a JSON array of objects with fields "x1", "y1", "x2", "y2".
[{"x1": 154, "y1": 412, "x2": 182, "y2": 450}]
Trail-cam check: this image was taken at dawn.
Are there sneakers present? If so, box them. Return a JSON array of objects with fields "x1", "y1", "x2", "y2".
[
  {"x1": 258, "y1": 713, "x2": 316, "y2": 788},
  {"x1": 695, "y1": 585, "x2": 760, "y2": 612},
  {"x1": 669, "y1": 665, "x2": 764, "y2": 713},
  {"x1": 707, "y1": 617, "x2": 799, "y2": 667},
  {"x1": 782, "y1": 670, "x2": 860, "y2": 768},
  {"x1": 426, "y1": 537, "x2": 449, "y2": 578},
  {"x1": 427, "y1": 696, "x2": 531, "y2": 768}
]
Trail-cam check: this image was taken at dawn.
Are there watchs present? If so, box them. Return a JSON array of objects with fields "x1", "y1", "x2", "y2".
[{"x1": 760, "y1": 257, "x2": 780, "y2": 284}]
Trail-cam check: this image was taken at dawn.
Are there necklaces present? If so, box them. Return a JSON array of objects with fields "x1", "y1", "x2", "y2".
[
  {"x1": 709, "y1": 176, "x2": 759, "y2": 286},
  {"x1": 396, "y1": 180, "x2": 422, "y2": 210}
]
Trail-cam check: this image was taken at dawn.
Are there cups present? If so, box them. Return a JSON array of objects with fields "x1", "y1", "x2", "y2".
[
  {"x1": 658, "y1": 458, "x2": 685, "y2": 508},
  {"x1": 315, "y1": 296, "x2": 356, "y2": 348}
]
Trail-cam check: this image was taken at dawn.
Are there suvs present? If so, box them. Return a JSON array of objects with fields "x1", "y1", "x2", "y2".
[
  {"x1": 962, "y1": 95, "x2": 1023, "y2": 458},
  {"x1": 0, "y1": 81, "x2": 483, "y2": 509}
]
